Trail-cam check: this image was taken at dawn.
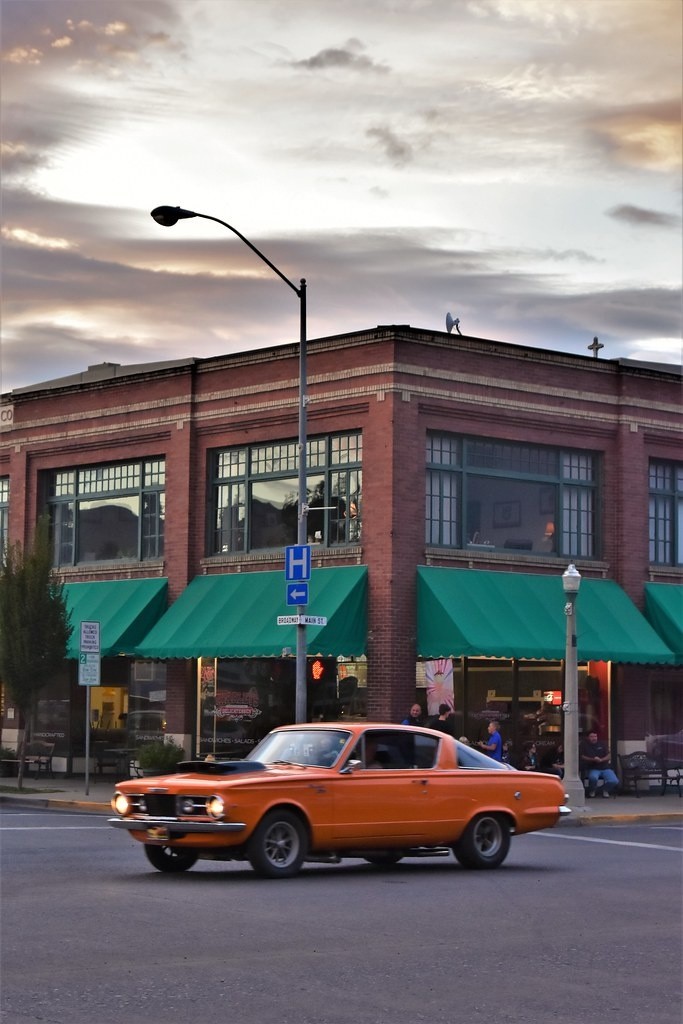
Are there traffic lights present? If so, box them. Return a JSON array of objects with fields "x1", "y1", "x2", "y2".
[{"x1": 308, "y1": 655, "x2": 337, "y2": 685}]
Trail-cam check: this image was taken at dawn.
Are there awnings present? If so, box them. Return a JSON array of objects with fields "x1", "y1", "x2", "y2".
[
  {"x1": 132, "y1": 565, "x2": 368, "y2": 660},
  {"x1": 643, "y1": 582, "x2": 683, "y2": 667},
  {"x1": 47, "y1": 576, "x2": 168, "y2": 659},
  {"x1": 415, "y1": 565, "x2": 675, "y2": 664}
]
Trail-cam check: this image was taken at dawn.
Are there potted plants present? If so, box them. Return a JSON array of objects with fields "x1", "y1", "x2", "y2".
[{"x1": 131, "y1": 736, "x2": 185, "y2": 777}]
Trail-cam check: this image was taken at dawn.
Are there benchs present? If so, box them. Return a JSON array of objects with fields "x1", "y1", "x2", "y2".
[
  {"x1": 1, "y1": 740, "x2": 56, "y2": 780},
  {"x1": 93, "y1": 739, "x2": 127, "y2": 784},
  {"x1": 617, "y1": 748, "x2": 683, "y2": 799}
]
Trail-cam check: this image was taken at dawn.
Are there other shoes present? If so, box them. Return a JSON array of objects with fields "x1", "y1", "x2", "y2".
[
  {"x1": 602, "y1": 789, "x2": 609, "y2": 798},
  {"x1": 590, "y1": 791, "x2": 596, "y2": 797}
]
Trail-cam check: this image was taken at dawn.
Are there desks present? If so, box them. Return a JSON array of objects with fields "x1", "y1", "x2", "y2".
[{"x1": 104, "y1": 748, "x2": 137, "y2": 783}]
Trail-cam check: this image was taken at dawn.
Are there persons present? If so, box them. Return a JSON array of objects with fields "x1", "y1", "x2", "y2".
[
  {"x1": 476, "y1": 721, "x2": 502, "y2": 762},
  {"x1": 579, "y1": 731, "x2": 619, "y2": 797},
  {"x1": 424, "y1": 704, "x2": 454, "y2": 736},
  {"x1": 546, "y1": 745, "x2": 564, "y2": 778},
  {"x1": 400, "y1": 703, "x2": 423, "y2": 726},
  {"x1": 522, "y1": 742, "x2": 540, "y2": 772}
]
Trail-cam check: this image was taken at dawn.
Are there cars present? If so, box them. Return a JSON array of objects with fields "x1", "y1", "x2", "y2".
[{"x1": 106, "y1": 720, "x2": 573, "y2": 879}]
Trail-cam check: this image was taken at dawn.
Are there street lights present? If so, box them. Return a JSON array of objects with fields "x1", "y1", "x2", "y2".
[
  {"x1": 148, "y1": 204, "x2": 313, "y2": 726},
  {"x1": 562, "y1": 563, "x2": 583, "y2": 809}
]
